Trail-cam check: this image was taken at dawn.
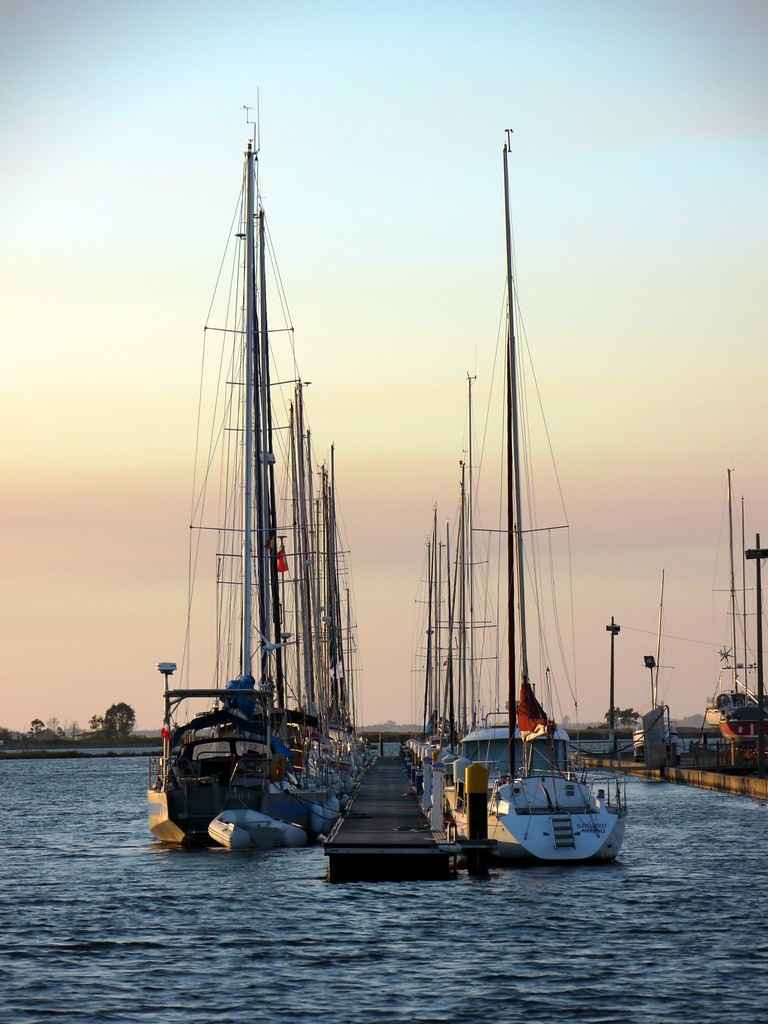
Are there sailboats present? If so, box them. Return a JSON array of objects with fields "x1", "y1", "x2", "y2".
[
  {"x1": 718, "y1": 497, "x2": 768, "y2": 747},
  {"x1": 632, "y1": 568, "x2": 679, "y2": 762},
  {"x1": 144, "y1": 86, "x2": 372, "y2": 851},
  {"x1": 705, "y1": 469, "x2": 754, "y2": 733},
  {"x1": 411, "y1": 129, "x2": 628, "y2": 866}
]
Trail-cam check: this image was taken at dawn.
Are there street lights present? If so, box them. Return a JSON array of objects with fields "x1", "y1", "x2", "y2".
[
  {"x1": 606, "y1": 617, "x2": 621, "y2": 752},
  {"x1": 745, "y1": 533, "x2": 768, "y2": 779},
  {"x1": 644, "y1": 656, "x2": 656, "y2": 711}
]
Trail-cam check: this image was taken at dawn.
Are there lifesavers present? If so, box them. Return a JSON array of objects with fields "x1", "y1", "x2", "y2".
[{"x1": 267, "y1": 752, "x2": 286, "y2": 784}]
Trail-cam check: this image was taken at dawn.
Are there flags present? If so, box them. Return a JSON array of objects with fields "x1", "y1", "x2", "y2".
[
  {"x1": 265, "y1": 536, "x2": 289, "y2": 572},
  {"x1": 161, "y1": 715, "x2": 172, "y2": 741},
  {"x1": 443, "y1": 660, "x2": 448, "y2": 666}
]
